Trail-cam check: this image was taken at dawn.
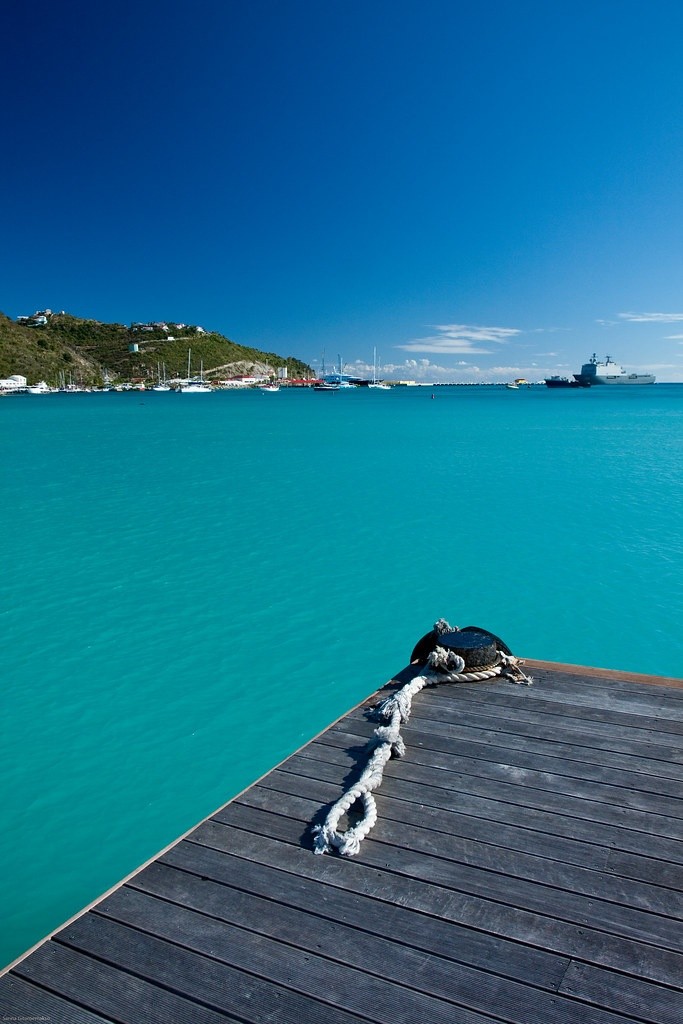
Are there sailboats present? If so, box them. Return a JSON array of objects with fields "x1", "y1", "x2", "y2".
[
  {"x1": 175, "y1": 348, "x2": 212, "y2": 393},
  {"x1": 367, "y1": 345, "x2": 391, "y2": 390},
  {"x1": 57, "y1": 369, "x2": 84, "y2": 393},
  {"x1": 153, "y1": 361, "x2": 171, "y2": 391}
]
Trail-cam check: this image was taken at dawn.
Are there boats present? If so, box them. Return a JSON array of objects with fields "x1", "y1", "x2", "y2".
[
  {"x1": 329, "y1": 358, "x2": 357, "y2": 389},
  {"x1": 24, "y1": 381, "x2": 53, "y2": 395},
  {"x1": 536, "y1": 380, "x2": 546, "y2": 385},
  {"x1": 515, "y1": 378, "x2": 528, "y2": 385},
  {"x1": 544, "y1": 374, "x2": 591, "y2": 389},
  {"x1": 313, "y1": 385, "x2": 341, "y2": 391},
  {"x1": 103, "y1": 388, "x2": 110, "y2": 392},
  {"x1": 572, "y1": 353, "x2": 656, "y2": 385},
  {"x1": 259, "y1": 383, "x2": 280, "y2": 392},
  {"x1": 506, "y1": 382, "x2": 519, "y2": 390}
]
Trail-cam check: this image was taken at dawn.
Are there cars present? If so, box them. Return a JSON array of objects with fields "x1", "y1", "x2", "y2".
[{"x1": 118, "y1": 382, "x2": 133, "y2": 390}]
